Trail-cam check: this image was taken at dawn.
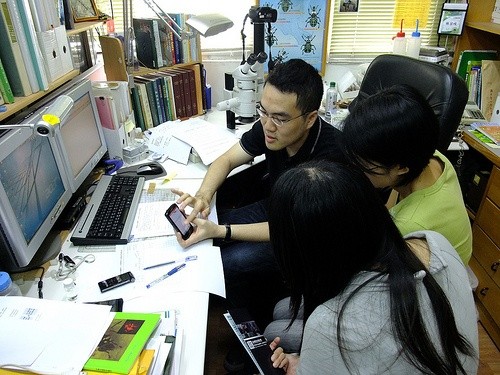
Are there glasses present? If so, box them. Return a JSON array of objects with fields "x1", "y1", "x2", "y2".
[{"x1": 256, "y1": 103, "x2": 318, "y2": 127}]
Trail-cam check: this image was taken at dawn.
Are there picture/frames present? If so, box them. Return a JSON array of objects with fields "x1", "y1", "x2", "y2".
[
  {"x1": 254, "y1": 0, "x2": 332, "y2": 78},
  {"x1": 69, "y1": 0, "x2": 100, "y2": 24}
]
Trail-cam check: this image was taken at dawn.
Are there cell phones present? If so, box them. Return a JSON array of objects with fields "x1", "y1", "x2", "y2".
[
  {"x1": 98, "y1": 271, "x2": 135, "y2": 292},
  {"x1": 165, "y1": 203, "x2": 194, "y2": 240}
]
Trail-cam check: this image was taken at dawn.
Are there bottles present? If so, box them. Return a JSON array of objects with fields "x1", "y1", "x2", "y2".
[
  {"x1": 0, "y1": 271, "x2": 22, "y2": 296},
  {"x1": 406, "y1": 18, "x2": 422, "y2": 59},
  {"x1": 392, "y1": 19, "x2": 406, "y2": 56},
  {"x1": 325, "y1": 82, "x2": 338, "y2": 119}
]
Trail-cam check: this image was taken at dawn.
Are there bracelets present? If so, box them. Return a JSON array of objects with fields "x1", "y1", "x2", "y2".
[{"x1": 224, "y1": 225, "x2": 231, "y2": 240}]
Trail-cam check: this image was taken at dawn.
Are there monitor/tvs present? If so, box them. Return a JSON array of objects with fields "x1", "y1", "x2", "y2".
[
  {"x1": 0, "y1": 114, "x2": 73, "y2": 272},
  {"x1": 35, "y1": 78, "x2": 107, "y2": 192}
]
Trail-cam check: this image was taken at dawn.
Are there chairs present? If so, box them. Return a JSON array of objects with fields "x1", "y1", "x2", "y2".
[{"x1": 346, "y1": 52, "x2": 469, "y2": 210}]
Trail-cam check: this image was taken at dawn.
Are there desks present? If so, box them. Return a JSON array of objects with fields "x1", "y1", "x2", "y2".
[
  {"x1": 214, "y1": 102, "x2": 468, "y2": 178},
  {"x1": 0, "y1": 104, "x2": 214, "y2": 375}
]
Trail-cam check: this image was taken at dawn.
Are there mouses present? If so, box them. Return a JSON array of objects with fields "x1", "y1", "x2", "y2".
[{"x1": 137, "y1": 163, "x2": 164, "y2": 175}]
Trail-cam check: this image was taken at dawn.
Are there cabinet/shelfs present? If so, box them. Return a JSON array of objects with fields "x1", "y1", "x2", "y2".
[
  {"x1": 0, "y1": 13, "x2": 112, "y2": 121},
  {"x1": 449, "y1": 0, "x2": 500, "y2": 354}
]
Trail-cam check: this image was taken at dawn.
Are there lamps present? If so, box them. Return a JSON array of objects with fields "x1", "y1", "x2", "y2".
[
  {"x1": 121, "y1": 0, "x2": 235, "y2": 77},
  {"x1": 0, "y1": 94, "x2": 75, "y2": 138}
]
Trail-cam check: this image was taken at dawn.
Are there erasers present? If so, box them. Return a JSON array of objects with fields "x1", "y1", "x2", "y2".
[{"x1": 186, "y1": 255, "x2": 197, "y2": 261}]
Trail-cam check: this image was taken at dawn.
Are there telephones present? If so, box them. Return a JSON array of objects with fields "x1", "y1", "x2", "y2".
[{"x1": 461, "y1": 101, "x2": 487, "y2": 124}]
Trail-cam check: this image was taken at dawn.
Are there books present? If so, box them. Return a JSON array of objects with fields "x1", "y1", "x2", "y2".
[
  {"x1": 457, "y1": 50, "x2": 497, "y2": 108},
  {"x1": 132, "y1": 13, "x2": 198, "y2": 69},
  {"x1": 131, "y1": 67, "x2": 198, "y2": 131},
  {"x1": 0, "y1": 0, "x2": 60, "y2": 103},
  {"x1": 83, "y1": 312, "x2": 162, "y2": 375}
]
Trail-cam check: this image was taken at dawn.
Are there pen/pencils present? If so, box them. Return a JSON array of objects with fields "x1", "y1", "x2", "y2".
[
  {"x1": 143, "y1": 261, "x2": 174, "y2": 270},
  {"x1": 146, "y1": 264, "x2": 185, "y2": 288}
]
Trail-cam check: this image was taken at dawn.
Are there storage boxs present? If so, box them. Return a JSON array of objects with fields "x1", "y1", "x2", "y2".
[
  {"x1": 418, "y1": 47, "x2": 448, "y2": 67},
  {"x1": 122, "y1": 141, "x2": 149, "y2": 157},
  {"x1": 122, "y1": 149, "x2": 151, "y2": 165},
  {"x1": 123, "y1": 108, "x2": 136, "y2": 146},
  {"x1": 101, "y1": 122, "x2": 128, "y2": 160}
]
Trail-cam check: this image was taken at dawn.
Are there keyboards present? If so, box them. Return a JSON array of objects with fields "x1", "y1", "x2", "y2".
[{"x1": 70, "y1": 174, "x2": 145, "y2": 247}]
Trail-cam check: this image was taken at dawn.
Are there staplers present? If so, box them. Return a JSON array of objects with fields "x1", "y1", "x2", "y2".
[{"x1": 103, "y1": 160, "x2": 123, "y2": 174}]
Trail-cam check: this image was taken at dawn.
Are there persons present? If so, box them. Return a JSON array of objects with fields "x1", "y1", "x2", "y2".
[
  {"x1": 270, "y1": 156, "x2": 480, "y2": 375},
  {"x1": 171, "y1": 58, "x2": 349, "y2": 314},
  {"x1": 264, "y1": 84, "x2": 472, "y2": 351}
]
